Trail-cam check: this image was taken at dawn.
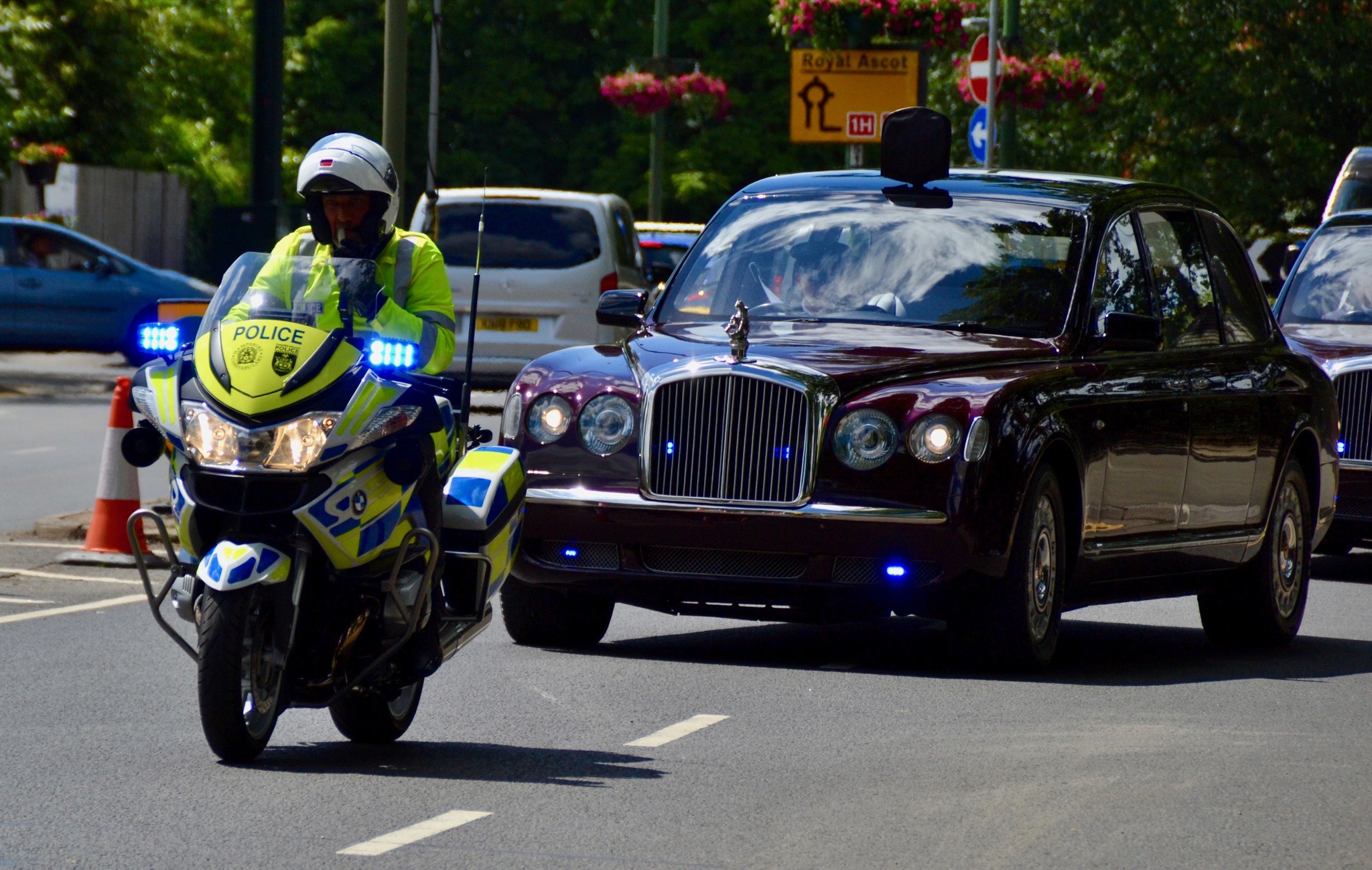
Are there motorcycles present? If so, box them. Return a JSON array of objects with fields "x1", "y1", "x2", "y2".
[{"x1": 119, "y1": 251, "x2": 527, "y2": 769}]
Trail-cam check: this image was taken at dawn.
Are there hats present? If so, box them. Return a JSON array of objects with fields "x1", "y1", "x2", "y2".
[{"x1": 790, "y1": 242, "x2": 850, "y2": 282}]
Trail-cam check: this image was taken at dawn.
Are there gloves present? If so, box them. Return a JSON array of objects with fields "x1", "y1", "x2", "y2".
[{"x1": 338, "y1": 260, "x2": 386, "y2": 317}]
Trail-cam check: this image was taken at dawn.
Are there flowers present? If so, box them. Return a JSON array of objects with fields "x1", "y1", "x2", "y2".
[
  {"x1": 602, "y1": 71, "x2": 731, "y2": 128},
  {"x1": 11, "y1": 140, "x2": 70, "y2": 165},
  {"x1": 767, "y1": 0, "x2": 976, "y2": 54},
  {"x1": 952, "y1": 55, "x2": 1105, "y2": 118}
]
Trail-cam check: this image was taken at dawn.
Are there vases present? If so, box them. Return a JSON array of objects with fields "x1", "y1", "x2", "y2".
[{"x1": 21, "y1": 162, "x2": 57, "y2": 186}]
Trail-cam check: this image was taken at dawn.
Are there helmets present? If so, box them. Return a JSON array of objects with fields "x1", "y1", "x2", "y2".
[{"x1": 297, "y1": 132, "x2": 399, "y2": 245}]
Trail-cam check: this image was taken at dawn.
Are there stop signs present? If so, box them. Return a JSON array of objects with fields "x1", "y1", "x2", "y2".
[{"x1": 969, "y1": 35, "x2": 1005, "y2": 103}]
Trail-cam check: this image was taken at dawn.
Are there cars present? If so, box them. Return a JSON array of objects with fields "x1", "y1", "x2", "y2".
[
  {"x1": 404, "y1": 187, "x2": 671, "y2": 394},
  {"x1": 491, "y1": 165, "x2": 1347, "y2": 666},
  {"x1": 1262, "y1": 210, "x2": 1372, "y2": 553},
  {"x1": 633, "y1": 232, "x2": 707, "y2": 287},
  {"x1": 0, "y1": 219, "x2": 217, "y2": 359}
]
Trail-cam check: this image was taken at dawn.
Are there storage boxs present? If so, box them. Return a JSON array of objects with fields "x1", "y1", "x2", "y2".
[
  {"x1": 442, "y1": 445, "x2": 528, "y2": 610},
  {"x1": 428, "y1": 394, "x2": 459, "y2": 475}
]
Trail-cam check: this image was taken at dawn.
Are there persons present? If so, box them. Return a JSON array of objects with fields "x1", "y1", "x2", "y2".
[{"x1": 216, "y1": 131, "x2": 454, "y2": 635}]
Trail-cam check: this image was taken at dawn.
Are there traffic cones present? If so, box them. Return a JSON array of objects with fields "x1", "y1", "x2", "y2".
[{"x1": 57, "y1": 379, "x2": 176, "y2": 571}]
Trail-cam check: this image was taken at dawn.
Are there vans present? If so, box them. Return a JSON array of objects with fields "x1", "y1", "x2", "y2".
[{"x1": 1320, "y1": 147, "x2": 1372, "y2": 224}]
